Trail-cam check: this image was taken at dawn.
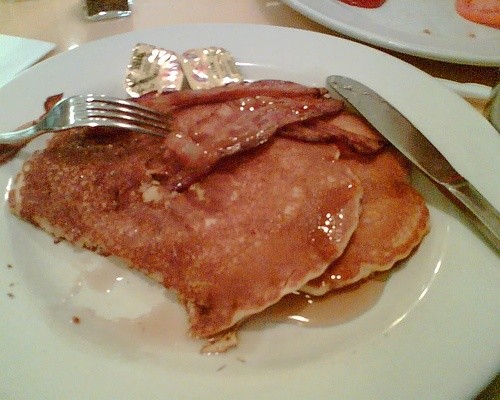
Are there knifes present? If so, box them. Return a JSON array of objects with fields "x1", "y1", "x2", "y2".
[{"x1": 328, "y1": 76, "x2": 500, "y2": 254}]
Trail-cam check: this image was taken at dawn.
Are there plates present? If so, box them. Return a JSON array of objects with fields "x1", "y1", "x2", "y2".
[
  {"x1": 0, "y1": 24, "x2": 500, "y2": 400},
  {"x1": 282, "y1": 0, "x2": 499, "y2": 66}
]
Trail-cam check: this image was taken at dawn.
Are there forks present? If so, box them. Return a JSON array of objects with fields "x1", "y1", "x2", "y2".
[{"x1": 0, "y1": 93, "x2": 173, "y2": 144}]
